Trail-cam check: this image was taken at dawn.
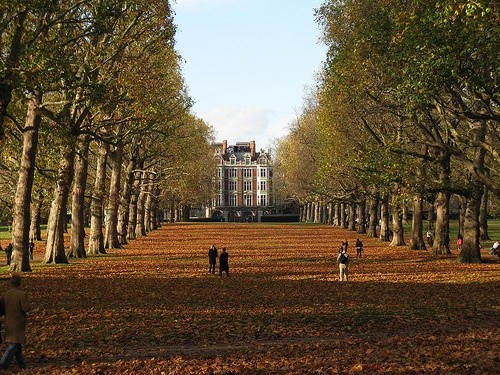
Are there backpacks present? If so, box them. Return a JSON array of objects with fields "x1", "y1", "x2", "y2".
[{"x1": 338, "y1": 254, "x2": 347, "y2": 264}]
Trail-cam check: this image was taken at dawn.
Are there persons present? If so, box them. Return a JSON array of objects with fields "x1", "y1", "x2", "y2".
[
  {"x1": 218, "y1": 247, "x2": 231, "y2": 276},
  {"x1": 0, "y1": 274, "x2": 31, "y2": 370},
  {"x1": 346, "y1": 214, "x2": 386, "y2": 230},
  {"x1": 336, "y1": 237, "x2": 350, "y2": 281},
  {"x1": 207, "y1": 243, "x2": 218, "y2": 274},
  {"x1": 425, "y1": 229, "x2": 500, "y2": 257},
  {"x1": 355, "y1": 239, "x2": 363, "y2": 256},
  {"x1": 28, "y1": 238, "x2": 35, "y2": 260},
  {"x1": 4, "y1": 243, "x2": 13, "y2": 266}
]
